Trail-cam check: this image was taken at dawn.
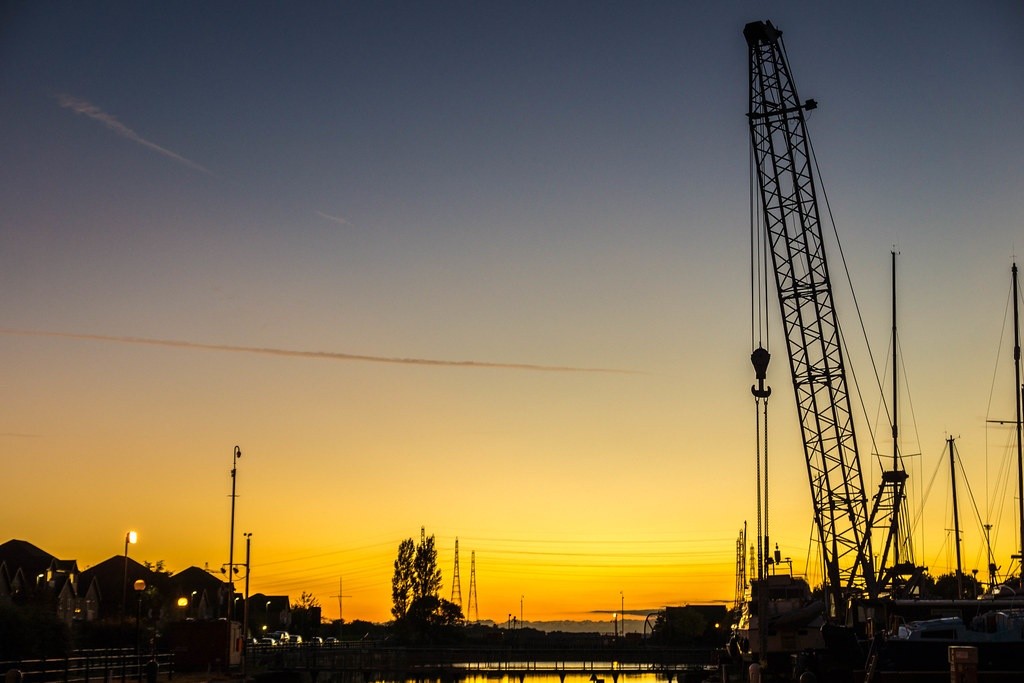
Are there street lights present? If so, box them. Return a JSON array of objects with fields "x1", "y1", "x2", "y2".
[
  {"x1": 146, "y1": 595, "x2": 189, "y2": 682},
  {"x1": 117, "y1": 531, "x2": 138, "y2": 650},
  {"x1": 242, "y1": 531, "x2": 253, "y2": 681},
  {"x1": 228, "y1": 445, "x2": 242, "y2": 619}
]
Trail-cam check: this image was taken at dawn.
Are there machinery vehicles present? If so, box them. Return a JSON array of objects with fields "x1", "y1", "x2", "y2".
[{"x1": 729, "y1": 12, "x2": 911, "y2": 683}]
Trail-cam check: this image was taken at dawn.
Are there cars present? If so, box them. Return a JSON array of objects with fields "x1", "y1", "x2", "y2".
[{"x1": 255, "y1": 630, "x2": 340, "y2": 648}]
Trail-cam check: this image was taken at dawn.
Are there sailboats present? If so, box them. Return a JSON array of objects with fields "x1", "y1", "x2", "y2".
[{"x1": 819, "y1": 241, "x2": 1024, "y2": 681}]
